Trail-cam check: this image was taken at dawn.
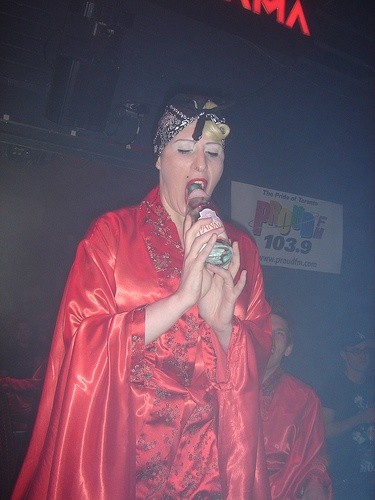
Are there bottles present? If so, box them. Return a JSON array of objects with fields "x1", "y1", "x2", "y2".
[{"x1": 187, "y1": 183, "x2": 233, "y2": 268}]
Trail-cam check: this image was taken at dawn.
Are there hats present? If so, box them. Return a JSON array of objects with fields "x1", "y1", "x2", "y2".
[
  {"x1": 339, "y1": 329, "x2": 373, "y2": 347},
  {"x1": 152, "y1": 94, "x2": 232, "y2": 156}
]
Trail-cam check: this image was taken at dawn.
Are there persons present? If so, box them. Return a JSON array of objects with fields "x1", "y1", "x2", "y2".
[
  {"x1": 7, "y1": 320, "x2": 45, "y2": 379},
  {"x1": 8, "y1": 94, "x2": 275, "y2": 499},
  {"x1": 324, "y1": 330, "x2": 375, "y2": 500},
  {"x1": 255, "y1": 312, "x2": 332, "y2": 500}
]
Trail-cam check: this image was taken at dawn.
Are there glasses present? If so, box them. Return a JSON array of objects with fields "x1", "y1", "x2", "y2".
[{"x1": 344, "y1": 348, "x2": 372, "y2": 354}]
